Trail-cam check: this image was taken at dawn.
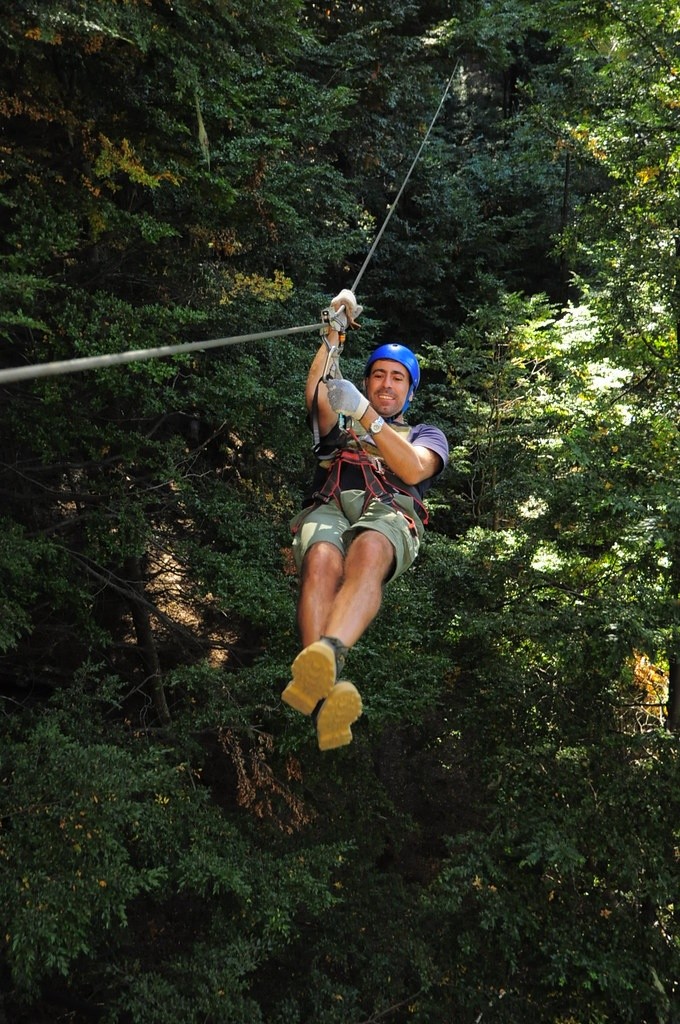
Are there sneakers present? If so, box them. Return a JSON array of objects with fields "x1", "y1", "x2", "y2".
[{"x1": 281, "y1": 635, "x2": 363, "y2": 750}]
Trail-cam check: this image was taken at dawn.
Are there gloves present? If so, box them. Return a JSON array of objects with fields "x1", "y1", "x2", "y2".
[
  {"x1": 326, "y1": 378, "x2": 371, "y2": 421},
  {"x1": 329, "y1": 288, "x2": 363, "y2": 331}
]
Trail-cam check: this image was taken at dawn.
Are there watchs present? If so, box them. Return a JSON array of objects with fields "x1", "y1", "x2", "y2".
[{"x1": 367, "y1": 416, "x2": 384, "y2": 437}]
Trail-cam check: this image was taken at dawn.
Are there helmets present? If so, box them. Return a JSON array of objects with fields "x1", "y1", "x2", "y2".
[{"x1": 363, "y1": 343, "x2": 420, "y2": 423}]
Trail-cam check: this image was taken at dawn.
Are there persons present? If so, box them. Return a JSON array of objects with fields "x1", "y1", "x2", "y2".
[{"x1": 281, "y1": 288, "x2": 449, "y2": 750}]
